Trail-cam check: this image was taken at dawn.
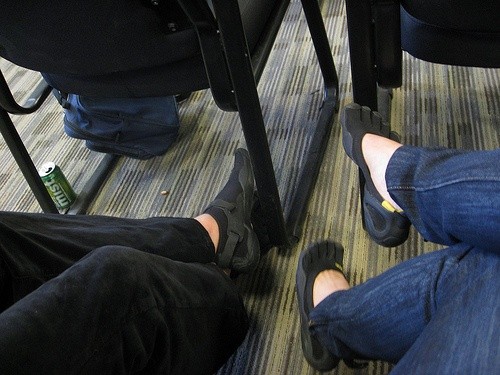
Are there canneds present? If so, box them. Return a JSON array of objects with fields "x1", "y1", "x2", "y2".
[{"x1": 37, "y1": 161, "x2": 76, "y2": 209}]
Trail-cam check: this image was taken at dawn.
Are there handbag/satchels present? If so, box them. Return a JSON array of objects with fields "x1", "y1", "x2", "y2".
[{"x1": 51, "y1": 87, "x2": 178, "y2": 160}]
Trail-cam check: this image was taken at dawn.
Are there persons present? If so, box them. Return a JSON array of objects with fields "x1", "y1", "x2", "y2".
[
  {"x1": 294, "y1": 102, "x2": 500, "y2": 375},
  {"x1": 0, "y1": 149, "x2": 261, "y2": 375}
]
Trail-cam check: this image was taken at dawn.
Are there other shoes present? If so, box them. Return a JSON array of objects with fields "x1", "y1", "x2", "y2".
[
  {"x1": 208, "y1": 148, "x2": 261, "y2": 271},
  {"x1": 339, "y1": 103, "x2": 410, "y2": 247},
  {"x1": 296, "y1": 239, "x2": 346, "y2": 371}
]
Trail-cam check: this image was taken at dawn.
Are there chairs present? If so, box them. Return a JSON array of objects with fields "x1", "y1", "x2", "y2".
[{"x1": 0, "y1": 0, "x2": 341, "y2": 258}]
